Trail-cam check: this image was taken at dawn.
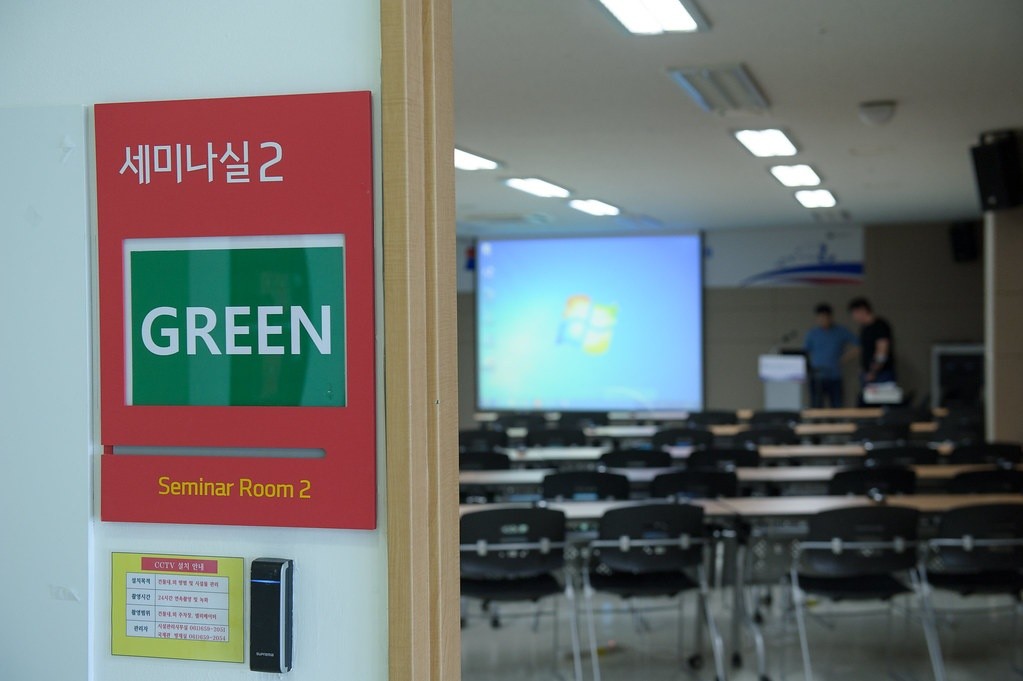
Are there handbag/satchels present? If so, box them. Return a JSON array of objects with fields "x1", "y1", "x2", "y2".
[{"x1": 863, "y1": 371, "x2": 904, "y2": 404}]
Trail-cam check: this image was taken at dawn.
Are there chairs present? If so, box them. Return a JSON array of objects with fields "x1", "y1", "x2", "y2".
[{"x1": 457, "y1": 409, "x2": 1023, "y2": 681}]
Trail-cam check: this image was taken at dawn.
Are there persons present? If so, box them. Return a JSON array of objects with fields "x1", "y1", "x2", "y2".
[
  {"x1": 849, "y1": 297, "x2": 897, "y2": 407},
  {"x1": 801, "y1": 303, "x2": 863, "y2": 409}
]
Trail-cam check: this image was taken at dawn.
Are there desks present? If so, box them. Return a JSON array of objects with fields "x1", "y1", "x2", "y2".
[{"x1": 457, "y1": 409, "x2": 1023, "y2": 632}]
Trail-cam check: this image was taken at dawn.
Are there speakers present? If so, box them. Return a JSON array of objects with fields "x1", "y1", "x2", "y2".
[
  {"x1": 949, "y1": 222, "x2": 984, "y2": 265},
  {"x1": 971, "y1": 133, "x2": 1023, "y2": 211}
]
